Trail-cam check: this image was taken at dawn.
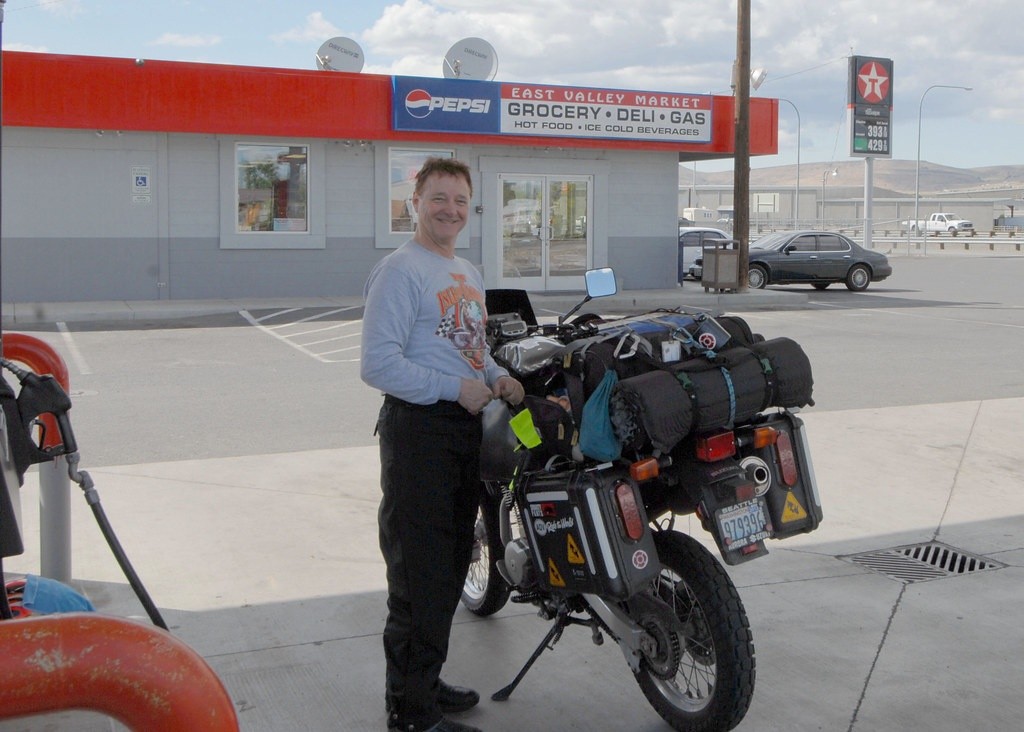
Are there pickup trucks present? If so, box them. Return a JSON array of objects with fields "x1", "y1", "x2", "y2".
[{"x1": 908, "y1": 212, "x2": 975, "y2": 235}]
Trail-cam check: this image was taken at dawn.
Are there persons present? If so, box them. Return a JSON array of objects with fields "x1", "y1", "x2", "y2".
[{"x1": 360, "y1": 157, "x2": 524, "y2": 732}]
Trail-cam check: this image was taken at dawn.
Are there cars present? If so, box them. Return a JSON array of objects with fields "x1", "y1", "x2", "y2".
[
  {"x1": 717, "y1": 218, "x2": 729, "y2": 224},
  {"x1": 679, "y1": 217, "x2": 695, "y2": 227},
  {"x1": 748, "y1": 229, "x2": 893, "y2": 292},
  {"x1": 678, "y1": 226, "x2": 734, "y2": 277}
]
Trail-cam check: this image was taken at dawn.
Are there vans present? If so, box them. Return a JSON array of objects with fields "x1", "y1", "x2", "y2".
[{"x1": 682, "y1": 206, "x2": 719, "y2": 224}]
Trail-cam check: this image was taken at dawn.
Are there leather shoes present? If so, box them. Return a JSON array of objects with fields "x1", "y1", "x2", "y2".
[
  {"x1": 437, "y1": 685, "x2": 480, "y2": 712},
  {"x1": 388, "y1": 716, "x2": 484, "y2": 732}
]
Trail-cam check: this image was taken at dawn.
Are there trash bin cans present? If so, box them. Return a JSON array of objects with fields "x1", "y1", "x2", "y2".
[{"x1": 701, "y1": 239, "x2": 740, "y2": 293}]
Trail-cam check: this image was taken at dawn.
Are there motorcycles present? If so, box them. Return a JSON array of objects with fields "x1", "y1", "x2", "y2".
[{"x1": 466, "y1": 265, "x2": 825, "y2": 732}]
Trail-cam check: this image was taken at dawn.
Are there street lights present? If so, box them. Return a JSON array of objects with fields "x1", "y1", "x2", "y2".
[
  {"x1": 916, "y1": 84, "x2": 974, "y2": 251},
  {"x1": 822, "y1": 167, "x2": 838, "y2": 231}
]
